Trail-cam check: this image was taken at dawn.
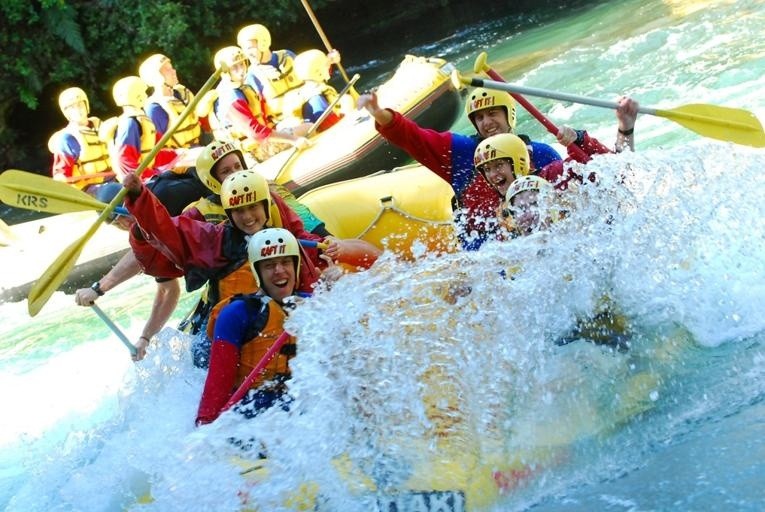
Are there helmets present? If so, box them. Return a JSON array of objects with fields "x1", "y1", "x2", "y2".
[
  {"x1": 504, "y1": 173, "x2": 561, "y2": 239},
  {"x1": 471, "y1": 132, "x2": 533, "y2": 197},
  {"x1": 194, "y1": 139, "x2": 249, "y2": 198},
  {"x1": 247, "y1": 226, "x2": 302, "y2": 292},
  {"x1": 464, "y1": 83, "x2": 517, "y2": 137},
  {"x1": 293, "y1": 48, "x2": 331, "y2": 84},
  {"x1": 57, "y1": 22, "x2": 273, "y2": 116},
  {"x1": 218, "y1": 168, "x2": 273, "y2": 233}
]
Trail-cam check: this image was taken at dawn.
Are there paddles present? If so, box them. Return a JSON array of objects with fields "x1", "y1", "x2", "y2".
[
  {"x1": 26, "y1": 61, "x2": 227, "y2": 316},
  {"x1": 451, "y1": 68, "x2": 765, "y2": 149},
  {"x1": 0, "y1": 170, "x2": 331, "y2": 251}
]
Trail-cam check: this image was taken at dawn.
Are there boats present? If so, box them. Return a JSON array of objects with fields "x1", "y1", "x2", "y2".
[
  {"x1": 145, "y1": 154, "x2": 705, "y2": 510},
  {"x1": 0, "y1": 48, "x2": 467, "y2": 305}
]
